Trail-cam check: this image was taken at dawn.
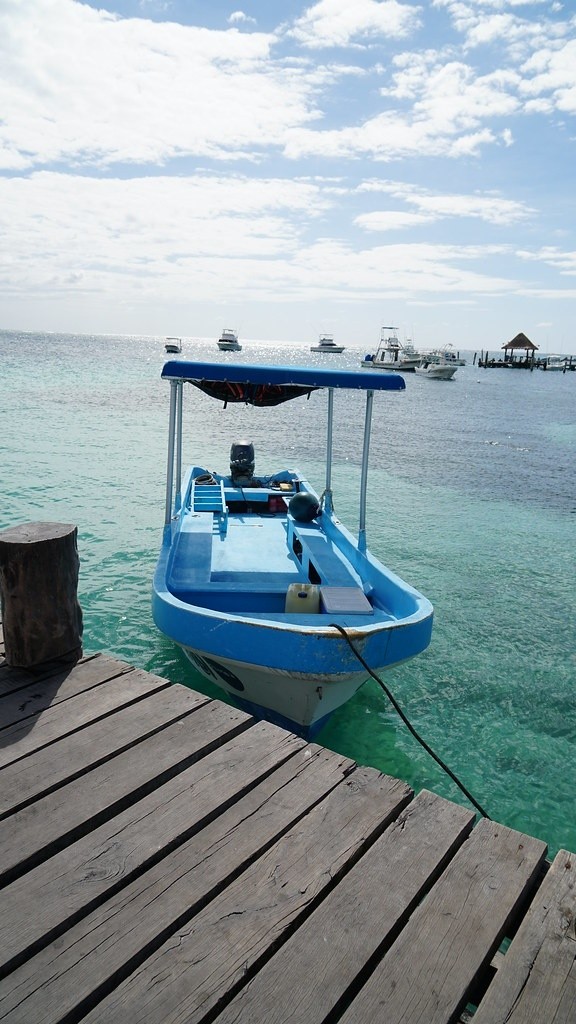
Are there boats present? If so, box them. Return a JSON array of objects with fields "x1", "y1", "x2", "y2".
[
  {"x1": 540, "y1": 355, "x2": 565, "y2": 369},
  {"x1": 310, "y1": 330, "x2": 345, "y2": 353},
  {"x1": 152, "y1": 360, "x2": 435, "y2": 741},
  {"x1": 165, "y1": 338, "x2": 182, "y2": 353},
  {"x1": 361, "y1": 320, "x2": 427, "y2": 368},
  {"x1": 216, "y1": 328, "x2": 242, "y2": 352},
  {"x1": 415, "y1": 343, "x2": 466, "y2": 381}
]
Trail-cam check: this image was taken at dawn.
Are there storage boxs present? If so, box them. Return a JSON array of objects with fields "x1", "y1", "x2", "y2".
[{"x1": 320, "y1": 587, "x2": 374, "y2": 615}]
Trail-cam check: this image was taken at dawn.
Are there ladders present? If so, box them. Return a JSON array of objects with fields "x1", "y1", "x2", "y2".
[{"x1": 191, "y1": 479, "x2": 229, "y2": 517}]
